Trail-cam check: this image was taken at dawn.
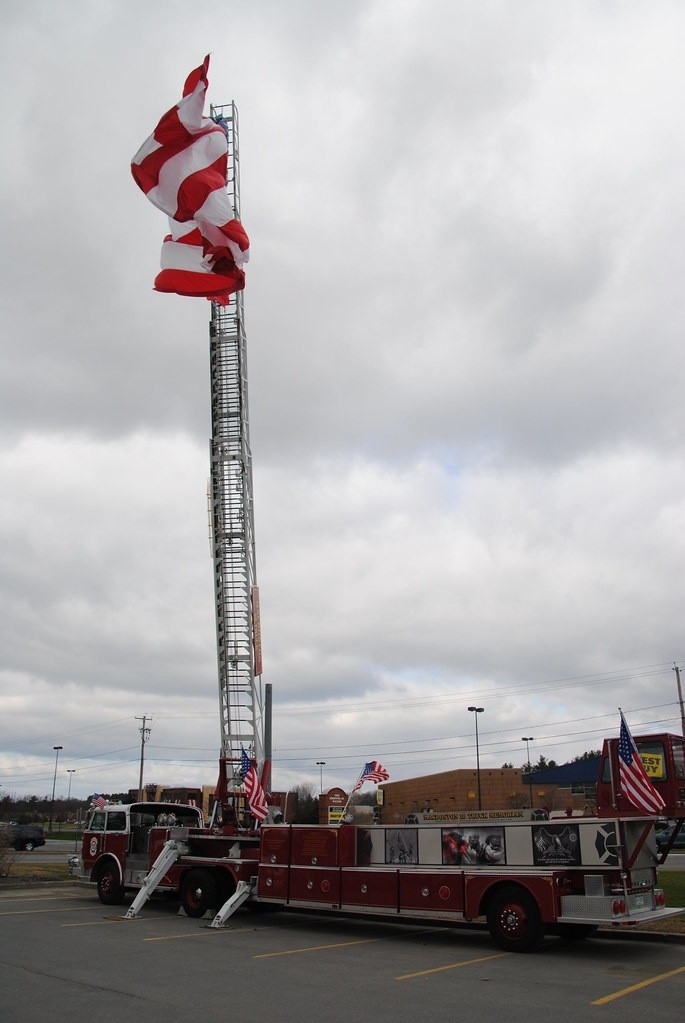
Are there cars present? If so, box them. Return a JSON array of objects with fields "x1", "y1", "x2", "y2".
[
  {"x1": 8, "y1": 824, "x2": 45, "y2": 851},
  {"x1": 655, "y1": 823, "x2": 684, "y2": 854}
]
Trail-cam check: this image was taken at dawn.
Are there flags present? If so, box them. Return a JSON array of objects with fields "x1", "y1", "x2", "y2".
[
  {"x1": 619, "y1": 712, "x2": 666, "y2": 816},
  {"x1": 129, "y1": 51, "x2": 250, "y2": 306},
  {"x1": 352, "y1": 761, "x2": 390, "y2": 794},
  {"x1": 92, "y1": 793, "x2": 107, "y2": 809},
  {"x1": 241, "y1": 747, "x2": 269, "y2": 824}
]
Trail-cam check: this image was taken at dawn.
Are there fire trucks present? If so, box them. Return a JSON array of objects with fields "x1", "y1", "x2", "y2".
[{"x1": 79, "y1": 98, "x2": 684, "y2": 953}]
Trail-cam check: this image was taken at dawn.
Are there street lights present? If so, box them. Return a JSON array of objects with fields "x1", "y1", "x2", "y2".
[
  {"x1": 65, "y1": 770, "x2": 75, "y2": 824},
  {"x1": 522, "y1": 737, "x2": 534, "y2": 809},
  {"x1": 468, "y1": 706, "x2": 486, "y2": 810},
  {"x1": 316, "y1": 762, "x2": 324, "y2": 794},
  {"x1": 48, "y1": 746, "x2": 63, "y2": 832}
]
